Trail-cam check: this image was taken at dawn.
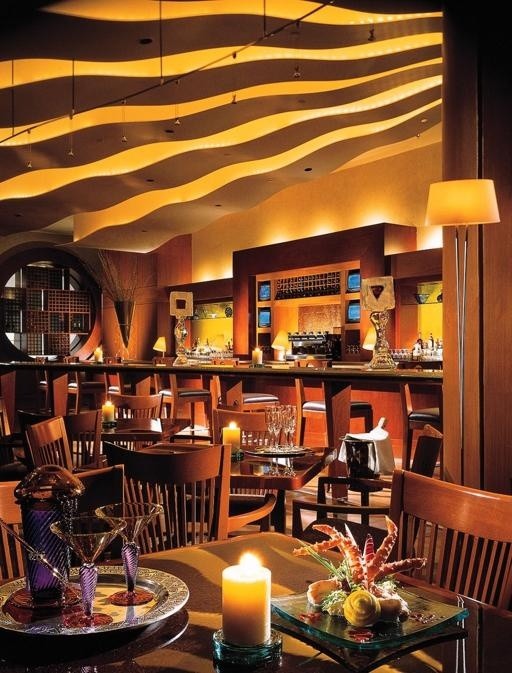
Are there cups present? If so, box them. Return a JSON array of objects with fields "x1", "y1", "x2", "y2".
[
  {"x1": 95, "y1": 502, "x2": 164, "y2": 606},
  {"x1": 290, "y1": 407, "x2": 297, "y2": 451},
  {"x1": 281, "y1": 404, "x2": 290, "y2": 452},
  {"x1": 390, "y1": 348, "x2": 411, "y2": 360},
  {"x1": 265, "y1": 406, "x2": 273, "y2": 451},
  {"x1": 272, "y1": 406, "x2": 282, "y2": 452},
  {"x1": 50, "y1": 515, "x2": 127, "y2": 628}
]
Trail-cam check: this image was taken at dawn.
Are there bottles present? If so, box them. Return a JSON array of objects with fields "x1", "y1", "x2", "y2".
[
  {"x1": 417, "y1": 329, "x2": 423, "y2": 349},
  {"x1": 428, "y1": 333, "x2": 433, "y2": 350}
]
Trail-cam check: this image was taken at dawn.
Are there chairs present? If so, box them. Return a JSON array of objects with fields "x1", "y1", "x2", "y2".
[
  {"x1": 399, "y1": 383, "x2": 442, "y2": 470},
  {"x1": 107, "y1": 392, "x2": 164, "y2": 450},
  {"x1": 0, "y1": 463, "x2": 124, "y2": 586},
  {"x1": 62, "y1": 410, "x2": 108, "y2": 470},
  {"x1": 213, "y1": 409, "x2": 294, "y2": 534},
  {"x1": 152, "y1": 357, "x2": 213, "y2": 437},
  {"x1": 35, "y1": 357, "x2": 53, "y2": 412},
  {"x1": 292, "y1": 425, "x2": 443, "y2": 575},
  {"x1": 294, "y1": 359, "x2": 373, "y2": 454},
  {"x1": 390, "y1": 470, "x2": 512, "y2": 622},
  {"x1": 103, "y1": 440, "x2": 231, "y2": 555},
  {"x1": 64, "y1": 356, "x2": 111, "y2": 415},
  {"x1": 209, "y1": 358, "x2": 280, "y2": 445},
  {"x1": 25, "y1": 416, "x2": 73, "y2": 476},
  {"x1": 104, "y1": 356, "x2": 135, "y2": 415}
]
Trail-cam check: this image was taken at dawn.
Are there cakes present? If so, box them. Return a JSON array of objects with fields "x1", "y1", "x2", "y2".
[{"x1": 342, "y1": 591, "x2": 381, "y2": 627}]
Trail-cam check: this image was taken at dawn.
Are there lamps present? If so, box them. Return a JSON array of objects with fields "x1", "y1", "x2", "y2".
[
  {"x1": 272, "y1": 330, "x2": 289, "y2": 365},
  {"x1": 424, "y1": 179, "x2": 501, "y2": 593},
  {"x1": 153, "y1": 336, "x2": 166, "y2": 357},
  {"x1": 363, "y1": 327, "x2": 378, "y2": 350}
]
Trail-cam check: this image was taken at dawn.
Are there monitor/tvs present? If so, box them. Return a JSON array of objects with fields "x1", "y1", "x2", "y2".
[
  {"x1": 347, "y1": 303, "x2": 360, "y2": 322},
  {"x1": 259, "y1": 281, "x2": 271, "y2": 301},
  {"x1": 258, "y1": 309, "x2": 271, "y2": 328},
  {"x1": 347, "y1": 273, "x2": 360, "y2": 292}
]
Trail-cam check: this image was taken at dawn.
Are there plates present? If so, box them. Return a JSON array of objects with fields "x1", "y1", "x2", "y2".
[
  {"x1": 271, "y1": 582, "x2": 469, "y2": 651},
  {"x1": 0, "y1": 566, "x2": 190, "y2": 636},
  {"x1": 246, "y1": 448, "x2": 307, "y2": 456}
]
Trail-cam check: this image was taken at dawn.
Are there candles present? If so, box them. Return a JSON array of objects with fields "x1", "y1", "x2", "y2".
[
  {"x1": 251, "y1": 350, "x2": 263, "y2": 366},
  {"x1": 223, "y1": 426, "x2": 240, "y2": 455},
  {"x1": 222, "y1": 553, "x2": 271, "y2": 647},
  {"x1": 102, "y1": 405, "x2": 115, "y2": 423},
  {"x1": 94, "y1": 351, "x2": 102, "y2": 362}
]
